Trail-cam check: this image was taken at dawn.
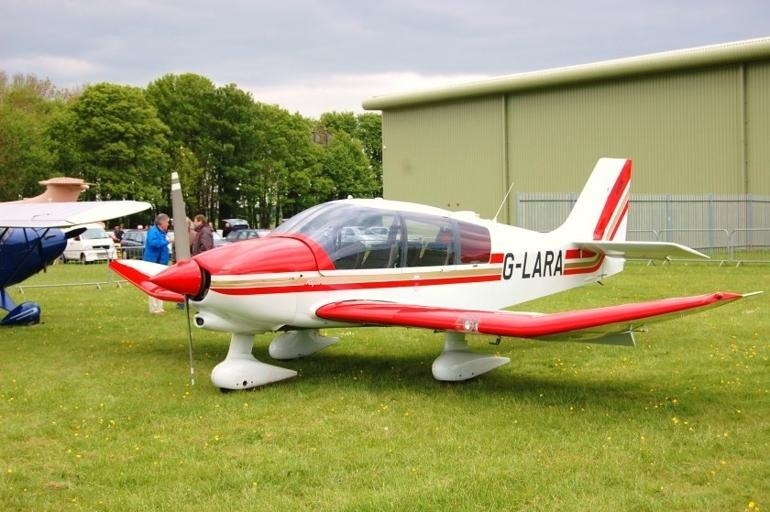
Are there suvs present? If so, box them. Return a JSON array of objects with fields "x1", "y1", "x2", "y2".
[
  {"x1": 368, "y1": 226, "x2": 423, "y2": 243},
  {"x1": 338, "y1": 226, "x2": 386, "y2": 245}
]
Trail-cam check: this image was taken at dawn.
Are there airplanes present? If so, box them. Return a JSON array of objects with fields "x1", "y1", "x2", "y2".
[
  {"x1": 108, "y1": 155, "x2": 765, "y2": 390},
  {"x1": 0, "y1": 176, "x2": 154, "y2": 325}
]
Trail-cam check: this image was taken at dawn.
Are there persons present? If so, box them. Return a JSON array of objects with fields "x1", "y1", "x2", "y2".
[
  {"x1": 171, "y1": 214, "x2": 206, "y2": 311},
  {"x1": 143, "y1": 213, "x2": 171, "y2": 314},
  {"x1": 190, "y1": 213, "x2": 215, "y2": 258},
  {"x1": 208, "y1": 221, "x2": 217, "y2": 232},
  {"x1": 111, "y1": 225, "x2": 125, "y2": 259}
]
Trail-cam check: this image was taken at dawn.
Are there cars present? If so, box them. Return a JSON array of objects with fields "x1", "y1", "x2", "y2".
[{"x1": 57, "y1": 218, "x2": 270, "y2": 264}]
